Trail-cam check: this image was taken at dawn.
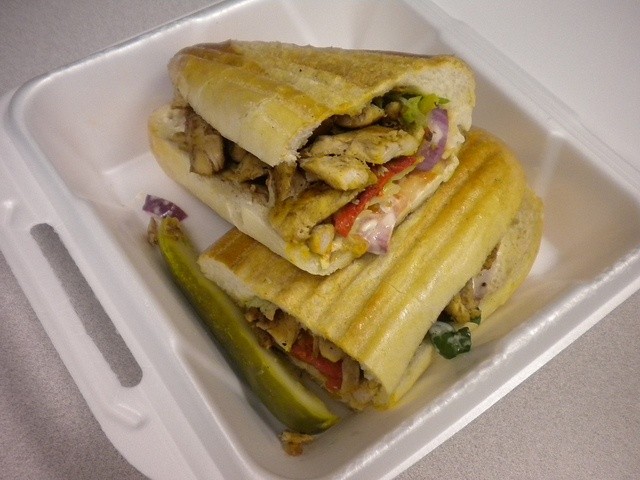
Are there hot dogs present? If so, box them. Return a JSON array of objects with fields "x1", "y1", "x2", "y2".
[
  {"x1": 196, "y1": 126, "x2": 546, "y2": 412},
  {"x1": 148, "y1": 38, "x2": 477, "y2": 276}
]
